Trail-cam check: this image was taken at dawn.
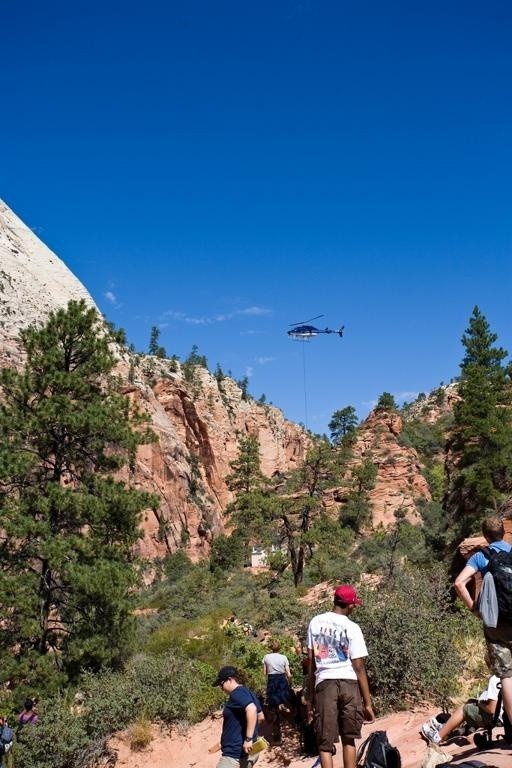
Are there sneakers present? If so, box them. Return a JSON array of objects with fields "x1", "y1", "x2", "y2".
[
  {"x1": 421, "y1": 723, "x2": 441, "y2": 744},
  {"x1": 431, "y1": 717, "x2": 443, "y2": 732},
  {"x1": 270, "y1": 739, "x2": 282, "y2": 745}
]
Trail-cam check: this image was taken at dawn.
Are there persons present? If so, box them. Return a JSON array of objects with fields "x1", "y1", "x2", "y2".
[
  {"x1": 304, "y1": 584, "x2": 377, "y2": 768},
  {"x1": 454, "y1": 516, "x2": 512, "y2": 750},
  {"x1": 20, "y1": 696, "x2": 41, "y2": 725},
  {"x1": 210, "y1": 638, "x2": 339, "y2": 768},
  {"x1": 420, "y1": 651, "x2": 504, "y2": 748},
  {"x1": 70, "y1": 692, "x2": 87, "y2": 716},
  {"x1": 0, "y1": 714, "x2": 14, "y2": 768}
]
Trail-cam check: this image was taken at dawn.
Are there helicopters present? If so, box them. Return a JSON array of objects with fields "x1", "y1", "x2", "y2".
[{"x1": 283, "y1": 312, "x2": 349, "y2": 342}]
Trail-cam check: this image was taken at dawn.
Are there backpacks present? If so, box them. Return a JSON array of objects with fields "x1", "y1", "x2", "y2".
[
  {"x1": 356, "y1": 730, "x2": 401, "y2": 767},
  {"x1": 298, "y1": 719, "x2": 337, "y2": 767},
  {"x1": 479, "y1": 544, "x2": 512, "y2": 617}
]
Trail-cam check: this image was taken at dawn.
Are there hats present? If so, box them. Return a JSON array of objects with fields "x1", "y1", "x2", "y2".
[
  {"x1": 211, "y1": 667, "x2": 239, "y2": 687},
  {"x1": 335, "y1": 583, "x2": 363, "y2": 607}
]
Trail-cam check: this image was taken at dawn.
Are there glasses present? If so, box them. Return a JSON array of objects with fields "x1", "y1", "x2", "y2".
[{"x1": 219, "y1": 681, "x2": 226, "y2": 691}]
